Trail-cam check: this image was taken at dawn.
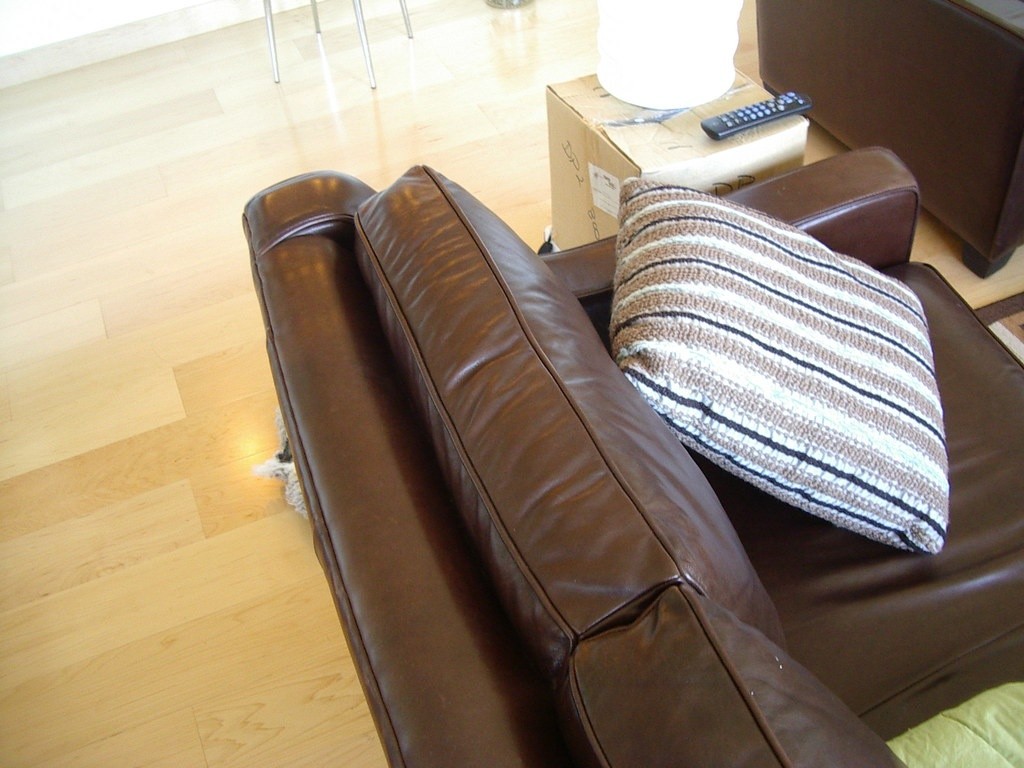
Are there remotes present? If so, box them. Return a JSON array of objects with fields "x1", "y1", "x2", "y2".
[{"x1": 700, "y1": 91, "x2": 813, "y2": 141}]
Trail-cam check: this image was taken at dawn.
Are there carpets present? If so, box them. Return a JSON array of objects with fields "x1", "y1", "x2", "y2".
[{"x1": 975, "y1": 290, "x2": 1024, "y2": 367}]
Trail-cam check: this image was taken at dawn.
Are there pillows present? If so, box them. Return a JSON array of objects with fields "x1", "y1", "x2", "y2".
[
  {"x1": 609, "y1": 179, "x2": 949, "y2": 556},
  {"x1": 880, "y1": 681, "x2": 1024, "y2": 768}
]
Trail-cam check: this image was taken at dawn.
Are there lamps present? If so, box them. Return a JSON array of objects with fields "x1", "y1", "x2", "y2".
[{"x1": 598, "y1": 0, "x2": 743, "y2": 108}]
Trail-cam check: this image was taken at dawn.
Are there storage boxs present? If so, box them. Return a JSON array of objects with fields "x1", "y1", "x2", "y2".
[{"x1": 545, "y1": 70, "x2": 810, "y2": 254}]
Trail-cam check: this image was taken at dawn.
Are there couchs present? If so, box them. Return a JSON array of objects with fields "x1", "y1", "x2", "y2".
[
  {"x1": 241, "y1": 146, "x2": 1024, "y2": 768},
  {"x1": 755, "y1": 0, "x2": 1024, "y2": 280}
]
